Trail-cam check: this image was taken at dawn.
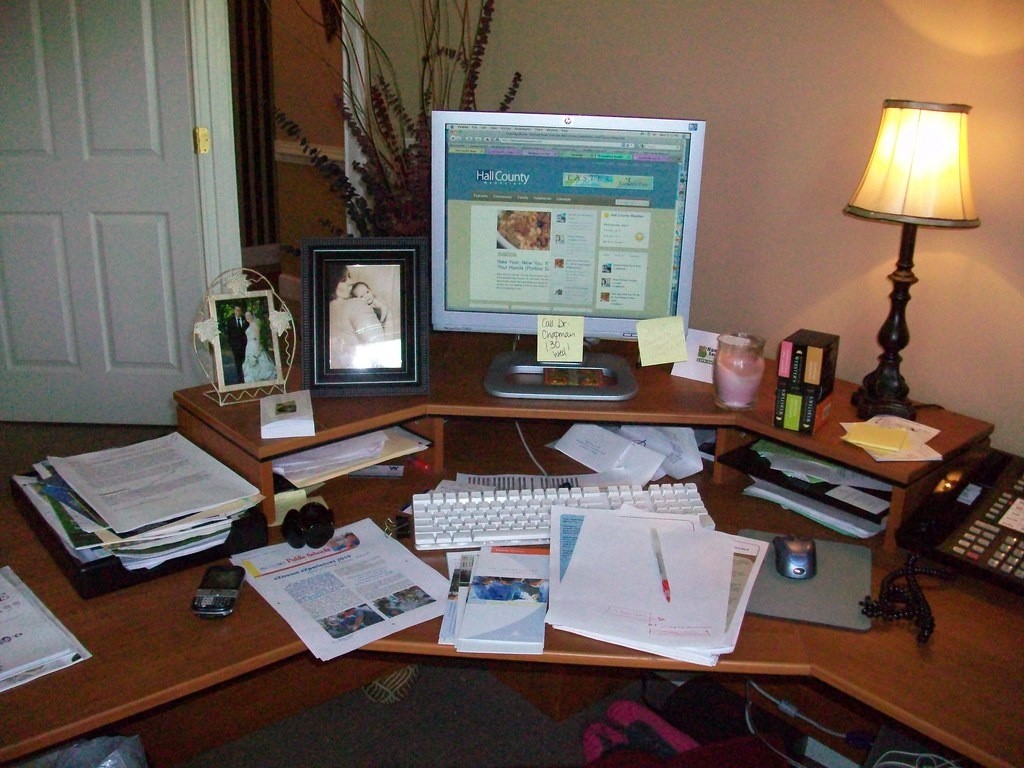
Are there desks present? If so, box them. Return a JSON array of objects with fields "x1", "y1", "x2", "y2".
[{"x1": 0, "y1": 332, "x2": 1024, "y2": 768}]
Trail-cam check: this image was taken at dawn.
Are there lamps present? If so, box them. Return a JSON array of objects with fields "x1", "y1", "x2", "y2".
[{"x1": 842, "y1": 100, "x2": 982, "y2": 421}]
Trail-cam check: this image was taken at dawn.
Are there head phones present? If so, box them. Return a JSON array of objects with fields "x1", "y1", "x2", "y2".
[{"x1": 282, "y1": 501, "x2": 335, "y2": 550}]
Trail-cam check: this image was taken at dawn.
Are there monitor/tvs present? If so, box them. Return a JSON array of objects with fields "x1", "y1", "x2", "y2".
[{"x1": 432, "y1": 111, "x2": 706, "y2": 401}]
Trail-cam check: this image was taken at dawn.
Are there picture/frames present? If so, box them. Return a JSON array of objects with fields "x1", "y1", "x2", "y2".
[
  {"x1": 208, "y1": 290, "x2": 285, "y2": 393},
  {"x1": 301, "y1": 236, "x2": 430, "y2": 398}
]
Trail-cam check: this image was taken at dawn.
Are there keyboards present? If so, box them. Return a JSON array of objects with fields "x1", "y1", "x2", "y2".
[{"x1": 412, "y1": 481, "x2": 716, "y2": 550}]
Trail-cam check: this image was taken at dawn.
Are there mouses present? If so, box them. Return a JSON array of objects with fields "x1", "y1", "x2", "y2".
[{"x1": 772, "y1": 536, "x2": 817, "y2": 579}]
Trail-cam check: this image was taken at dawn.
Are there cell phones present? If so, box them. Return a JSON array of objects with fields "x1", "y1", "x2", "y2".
[{"x1": 192, "y1": 565, "x2": 246, "y2": 617}]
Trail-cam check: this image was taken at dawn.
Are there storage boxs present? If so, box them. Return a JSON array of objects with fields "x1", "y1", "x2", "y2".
[{"x1": 773, "y1": 329, "x2": 840, "y2": 436}]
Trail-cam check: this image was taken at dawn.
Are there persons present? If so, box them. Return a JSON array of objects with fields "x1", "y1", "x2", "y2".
[
  {"x1": 311, "y1": 530, "x2": 549, "y2": 641},
  {"x1": 350, "y1": 281, "x2": 390, "y2": 328},
  {"x1": 226, "y1": 304, "x2": 249, "y2": 384},
  {"x1": 324, "y1": 264, "x2": 385, "y2": 348},
  {"x1": 241, "y1": 310, "x2": 277, "y2": 383}
]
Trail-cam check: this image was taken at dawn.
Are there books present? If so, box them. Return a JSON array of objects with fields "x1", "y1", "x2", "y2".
[{"x1": 0, "y1": 564, "x2": 92, "y2": 695}]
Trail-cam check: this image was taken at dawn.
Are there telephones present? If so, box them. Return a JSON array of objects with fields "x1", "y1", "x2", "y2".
[{"x1": 894, "y1": 444, "x2": 1024, "y2": 597}]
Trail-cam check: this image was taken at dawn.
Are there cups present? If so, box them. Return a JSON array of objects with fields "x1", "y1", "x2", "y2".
[{"x1": 711, "y1": 332, "x2": 767, "y2": 412}]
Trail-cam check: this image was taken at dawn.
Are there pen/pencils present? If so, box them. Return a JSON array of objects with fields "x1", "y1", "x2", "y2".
[{"x1": 650, "y1": 526, "x2": 671, "y2": 602}]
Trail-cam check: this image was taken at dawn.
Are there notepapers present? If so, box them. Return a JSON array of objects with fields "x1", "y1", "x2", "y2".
[{"x1": 842, "y1": 421, "x2": 912, "y2": 455}]
